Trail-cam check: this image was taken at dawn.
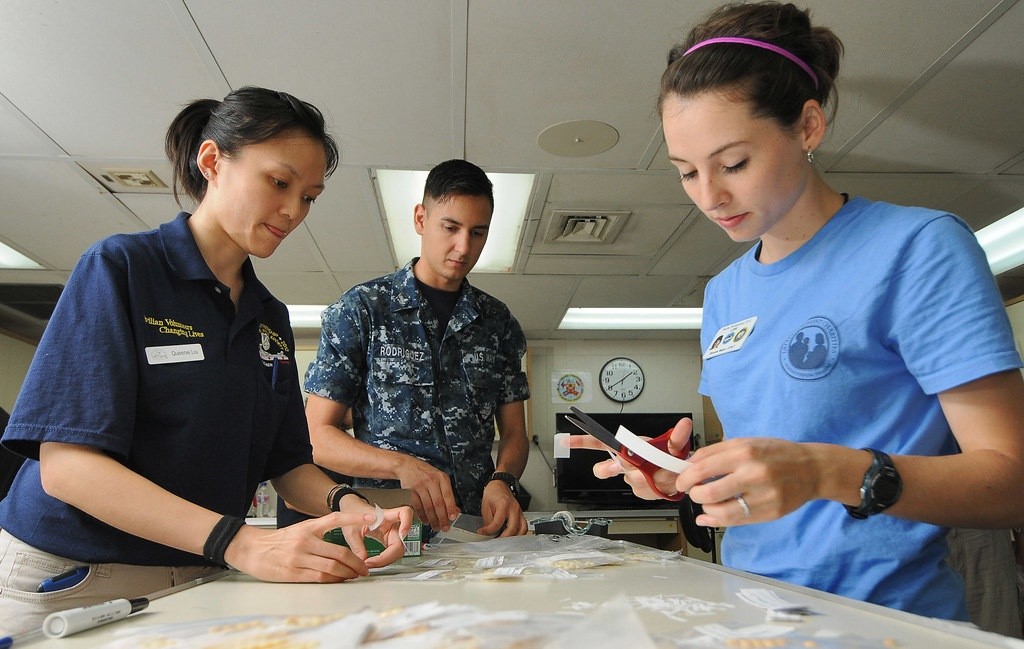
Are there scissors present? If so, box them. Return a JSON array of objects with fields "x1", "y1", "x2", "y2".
[{"x1": 564, "y1": 404, "x2": 693, "y2": 502}]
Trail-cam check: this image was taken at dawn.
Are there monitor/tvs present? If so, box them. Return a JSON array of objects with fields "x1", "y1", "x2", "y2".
[{"x1": 554, "y1": 412, "x2": 694, "y2": 510}]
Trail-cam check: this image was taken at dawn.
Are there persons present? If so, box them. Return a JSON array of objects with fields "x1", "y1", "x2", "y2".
[
  {"x1": 0, "y1": 87, "x2": 413, "y2": 641},
  {"x1": 943, "y1": 527, "x2": 1024, "y2": 641},
  {"x1": 277, "y1": 397, "x2": 354, "y2": 531},
  {"x1": 302, "y1": 158, "x2": 532, "y2": 539},
  {"x1": 558, "y1": 0, "x2": 1024, "y2": 622},
  {"x1": 0, "y1": 405, "x2": 27, "y2": 502}
]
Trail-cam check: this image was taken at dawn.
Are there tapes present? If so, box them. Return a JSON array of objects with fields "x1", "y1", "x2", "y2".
[{"x1": 552, "y1": 511, "x2": 576, "y2": 532}]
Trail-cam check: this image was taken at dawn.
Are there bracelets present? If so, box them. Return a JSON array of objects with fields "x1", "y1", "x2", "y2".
[
  {"x1": 326, "y1": 483, "x2": 369, "y2": 512},
  {"x1": 203, "y1": 515, "x2": 246, "y2": 565}
]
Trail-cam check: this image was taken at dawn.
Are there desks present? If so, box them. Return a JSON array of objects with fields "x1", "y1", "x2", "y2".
[{"x1": 0, "y1": 536, "x2": 1023, "y2": 649}]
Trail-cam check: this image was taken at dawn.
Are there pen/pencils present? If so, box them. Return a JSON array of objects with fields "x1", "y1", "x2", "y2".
[
  {"x1": 0, "y1": 626, "x2": 44, "y2": 649},
  {"x1": 271, "y1": 357, "x2": 279, "y2": 390}
]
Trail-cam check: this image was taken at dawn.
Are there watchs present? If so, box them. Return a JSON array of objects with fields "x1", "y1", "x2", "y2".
[
  {"x1": 482, "y1": 472, "x2": 520, "y2": 499},
  {"x1": 842, "y1": 448, "x2": 903, "y2": 519}
]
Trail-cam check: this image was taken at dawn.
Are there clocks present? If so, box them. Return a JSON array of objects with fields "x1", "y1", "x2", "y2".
[{"x1": 599, "y1": 357, "x2": 645, "y2": 403}]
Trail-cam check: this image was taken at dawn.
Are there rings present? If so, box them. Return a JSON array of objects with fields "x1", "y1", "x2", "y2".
[{"x1": 736, "y1": 496, "x2": 752, "y2": 518}]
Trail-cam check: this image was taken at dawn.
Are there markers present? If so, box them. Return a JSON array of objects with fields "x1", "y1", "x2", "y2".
[{"x1": 43, "y1": 592, "x2": 149, "y2": 636}]
